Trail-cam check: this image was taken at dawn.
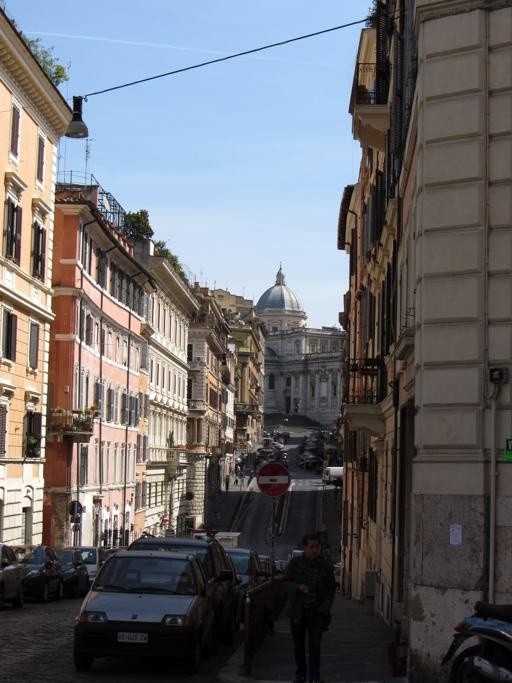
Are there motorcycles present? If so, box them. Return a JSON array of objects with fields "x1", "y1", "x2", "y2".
[{"x1": 441, "y1": 601, "x2": 512, "y2": 683}]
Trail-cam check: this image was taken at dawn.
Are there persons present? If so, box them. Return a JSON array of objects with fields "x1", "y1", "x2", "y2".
[{"x1": 284, "y1": 532, "x2": 336, "y2": 681}]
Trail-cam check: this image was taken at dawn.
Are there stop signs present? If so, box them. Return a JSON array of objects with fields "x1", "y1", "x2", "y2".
[{"x1": 257, "y1": 463, "x2": 291, "y2": 497}]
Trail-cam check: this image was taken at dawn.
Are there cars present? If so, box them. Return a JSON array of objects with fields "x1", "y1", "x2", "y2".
[{"x1": 257, "y1": 426, "x2": 320, "y2": 467}]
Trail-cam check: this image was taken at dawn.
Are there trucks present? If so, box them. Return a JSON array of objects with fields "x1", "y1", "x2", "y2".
[{"x1": 323, "y1": 466, "x2": 343, "y2": 488}]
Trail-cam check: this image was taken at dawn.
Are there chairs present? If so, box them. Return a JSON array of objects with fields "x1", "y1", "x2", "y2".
[{"x1": 117, "y1": 566, "x2": 194, "y2": 596}]
